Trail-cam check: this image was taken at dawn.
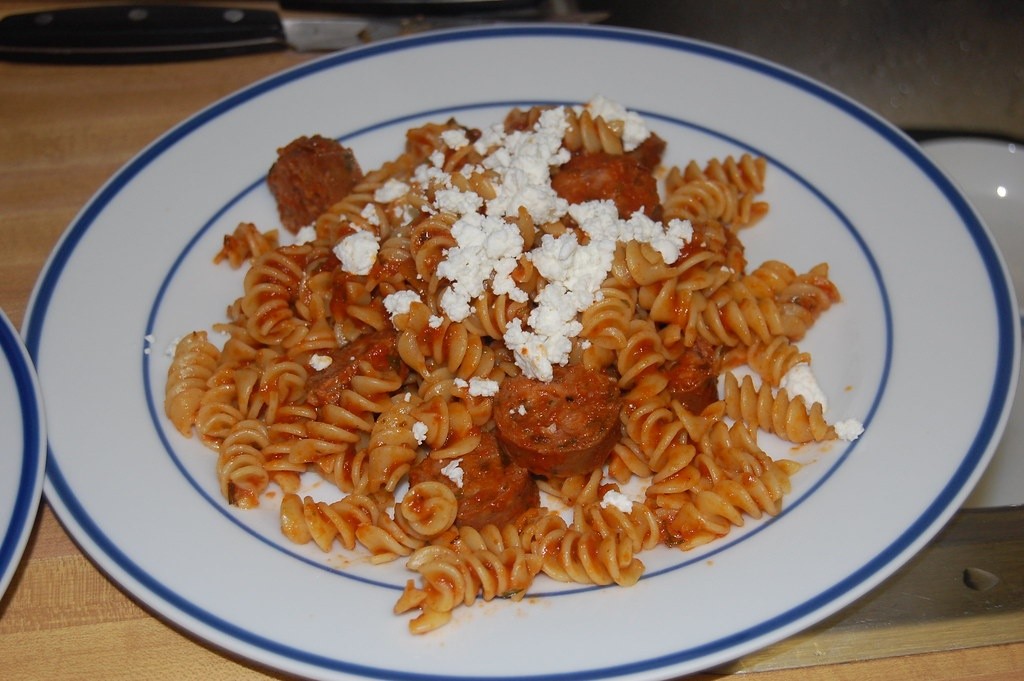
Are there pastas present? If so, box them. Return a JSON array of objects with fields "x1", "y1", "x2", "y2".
[{"x1": 165, "y1": 108, "x2": 840, "y2": 635}]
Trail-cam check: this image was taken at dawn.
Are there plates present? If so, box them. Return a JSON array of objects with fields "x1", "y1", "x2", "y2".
[
  {"x1": 0, "y1": 309, "x2": 47, "y2": 611},
  {"x1": 20, "y1": 21, "x2": 1021, "y2": 681},
  {"x1": 921, "y1": 137, "x2": 1024, "y2": 508}
]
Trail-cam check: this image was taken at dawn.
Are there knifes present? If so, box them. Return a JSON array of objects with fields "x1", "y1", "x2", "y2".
[{"x1": 0, "y1": 7, "x2": 611, "y2": 65}]
